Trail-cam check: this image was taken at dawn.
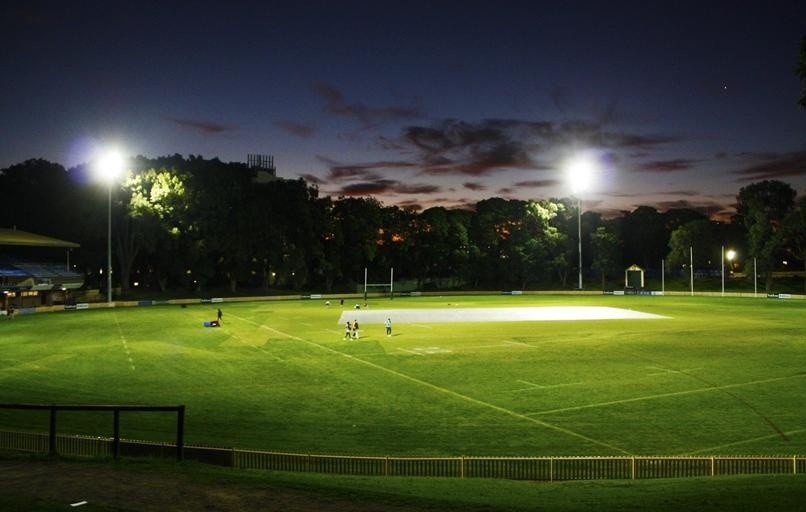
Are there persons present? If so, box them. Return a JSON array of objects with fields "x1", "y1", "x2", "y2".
[
  {"x1": 216, "y1": 308, "x2": 224, "y2": 325},
  {"x1": 384, "y1": 318, "x2": 392, "y2": 337},
  {"x1": 343, "y1": 319, "x2": 359, "y2": 342},
  {"x1": 6, "y1": 305, "x2": 15, "y2": 320}
]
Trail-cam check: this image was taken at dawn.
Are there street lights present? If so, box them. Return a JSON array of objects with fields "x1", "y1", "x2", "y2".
[
  {"x1": 563, "y1": 148, "x2": 593, "y2": 291},
  {"x1": 89, "y1": 145, "x2": 127, "y2": 304}
]
